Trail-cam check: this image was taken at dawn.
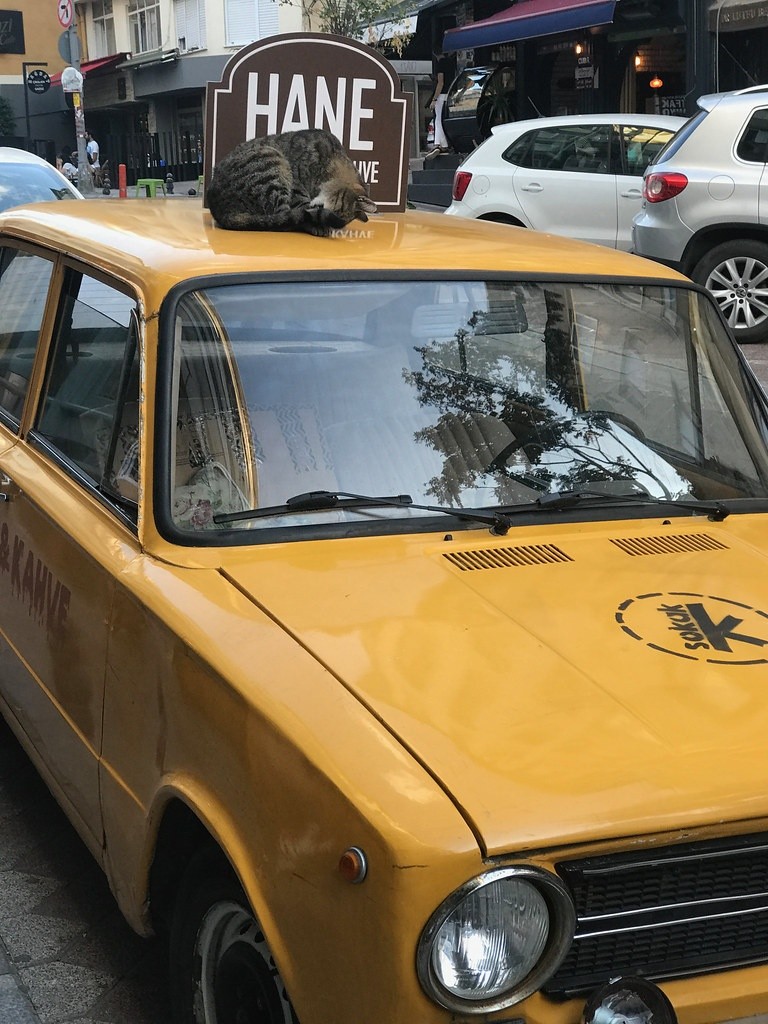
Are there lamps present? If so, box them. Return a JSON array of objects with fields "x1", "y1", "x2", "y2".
[{"x1": 648, "y1": 73, "x2": 663, "y2": 88}]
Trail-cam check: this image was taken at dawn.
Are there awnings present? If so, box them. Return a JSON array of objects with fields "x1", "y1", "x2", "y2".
[
  {"x1": 115, "y1": 48, "x2": 180, "y2": 70},
  {"x1": 50, "y1": 57, "x2": 117, "y2": 86},
  {"x1": 442, "y1": 1, "x2": 617, "y2": 53}
]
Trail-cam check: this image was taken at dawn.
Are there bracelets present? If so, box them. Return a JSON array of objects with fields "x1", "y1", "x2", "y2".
[{"x1": 432, "y1": 97, "x2": 437, "y2": 101}]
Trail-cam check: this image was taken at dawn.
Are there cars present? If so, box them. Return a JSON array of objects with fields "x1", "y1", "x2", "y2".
[{"x1": 0, "y1": 146, "x2": 86, "y2": 214}]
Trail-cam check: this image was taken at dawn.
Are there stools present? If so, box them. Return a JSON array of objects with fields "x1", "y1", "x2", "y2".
[
  {"x1": 135, "y1": 178, "x2": 167, "y2": 198},
  {"x1": 195, "y1": 175, "x2": 203, "y2": 196}
]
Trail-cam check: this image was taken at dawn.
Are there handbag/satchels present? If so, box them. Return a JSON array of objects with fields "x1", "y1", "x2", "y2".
[{"x1": 174, "y1": 462, "x2": 253, "y2": 529}]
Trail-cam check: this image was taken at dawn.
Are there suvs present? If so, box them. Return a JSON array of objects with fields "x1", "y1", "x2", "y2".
[
  {"x1": 444, "y1": 114, "x2": 691, "y2": 255},
  {"x1": 628, "y1": 83, "x2": 768, "y2": 344},
  {"x1": 0, "y1": 196, "x2": 768, "y2": 1024}
]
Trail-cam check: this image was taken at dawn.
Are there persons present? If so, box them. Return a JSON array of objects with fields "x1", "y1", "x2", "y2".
[
  {"x1": 424, "y1": 44, "x2": 456, "y2": 162},
  {"x1": 57, "y1": 129, "x2": 100, "y2": 177}
]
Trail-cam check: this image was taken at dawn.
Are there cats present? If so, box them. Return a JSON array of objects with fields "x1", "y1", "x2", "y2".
[{"x1": 206, "y1": 129, "x2": 376, "y2": 237}]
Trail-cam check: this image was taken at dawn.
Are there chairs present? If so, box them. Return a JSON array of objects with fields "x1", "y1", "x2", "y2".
[
  {"x1": 164, "y1": 397, "x2": 348, "y2": 522},
  {"x1": 352, "y1": 405, "x2": 547, "y2": 511}
]
